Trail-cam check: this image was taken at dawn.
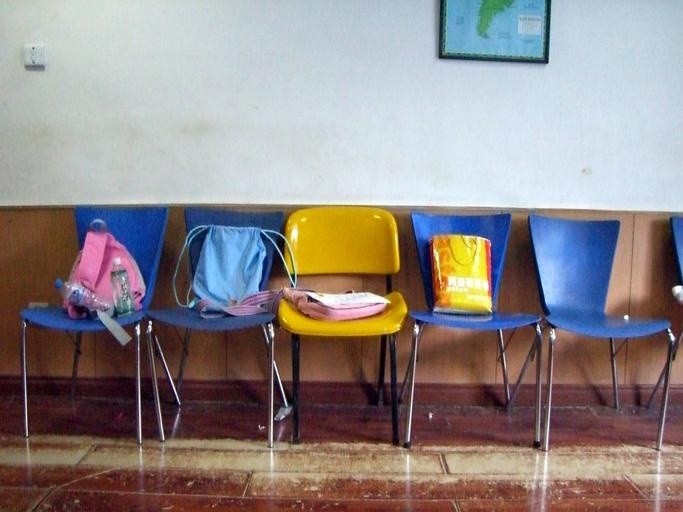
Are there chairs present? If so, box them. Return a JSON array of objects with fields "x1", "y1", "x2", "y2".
[
  {"x1": 644, "y1": 212, "x2": 683, "y2": 411},
  {"x1": 18, "y1": 203, "x2": 182, "y2": 446},
  {"x1": 277, "y1": 204, "x2": 407, "y2": 447},
  {"x1": 397, "y1": 210, "x2": 545, "y2": 450},
  {"x1": 509, "y1": 213, "x2": 678, "y2": 453},
  {"x1": 146, "y1": 206, "x2": 293, "y2": 447}
]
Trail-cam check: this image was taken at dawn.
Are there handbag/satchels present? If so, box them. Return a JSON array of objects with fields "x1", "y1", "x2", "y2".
[
  {"x1": 62, "y1": 218, "x2": 146, "y2": 346},
  {"x1": 430, "y1": 235, "x2": 493, "y2": 315},
  {"x1": 282, "y1": 284, "x2": 391, "y2": 320}
]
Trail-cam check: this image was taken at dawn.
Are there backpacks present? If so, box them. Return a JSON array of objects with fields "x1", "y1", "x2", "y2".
[{"x1": 172, "y1": 224, "x2": 298, "y2": 313}]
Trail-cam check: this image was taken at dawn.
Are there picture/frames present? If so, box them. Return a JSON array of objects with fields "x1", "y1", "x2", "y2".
[{"x1": 439, "y1": 0, "x2": 552, "y2": 65}]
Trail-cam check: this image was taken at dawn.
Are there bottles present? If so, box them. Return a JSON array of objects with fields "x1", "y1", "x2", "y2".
[
  {"x1": 52, "y1": 277, "x2": 113, "y2": 311},
  {"x1": 109, "y1": 257, "x2": 134, "y2": 315}
]
